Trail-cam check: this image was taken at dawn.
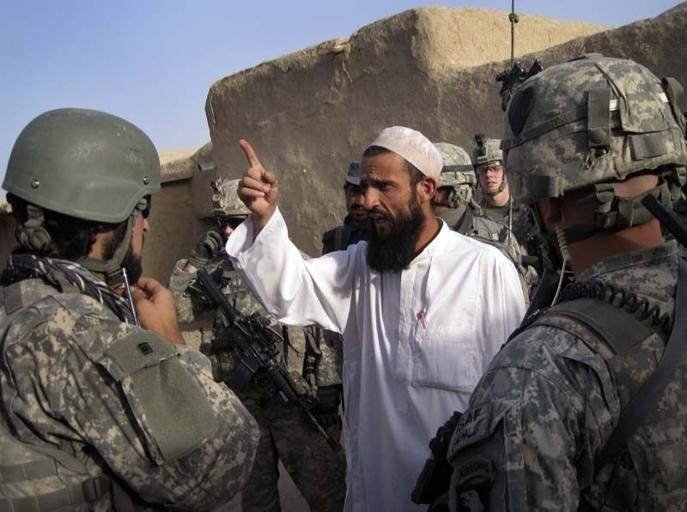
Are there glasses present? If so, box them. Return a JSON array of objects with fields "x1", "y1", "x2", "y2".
[
  {"x1": 136, "y1": 194, "x2": 157, "y2": 218},
  {"x1": 214, "y1": 216, "x2": 247, "y2": 228},
  {"x1": 474, "y1": 161, "x2": 504, "y2": 174}
]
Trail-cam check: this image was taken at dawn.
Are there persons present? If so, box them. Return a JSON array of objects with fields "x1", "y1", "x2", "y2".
[
  {"x1": 186, "y1": 176, "x2": 347, "y2": 512},
  {"x1": 304, "y1": 161, "x2": 376, "y2": 455},
  {"x1": 223, "y1": 123, "x2": 528, "y2": 511},
  {"x1": 430, "y1": 144, "x2": 537, "y2": 326},
  {"x1": 1, "y1": 107, "x2": 259, "y2": 512},
  {"x1": 472, "y1": 133, "x2": 556, "y2": 317},
  {"x1": 409, "y1": 54, "x2": 687, "y2": 510}
]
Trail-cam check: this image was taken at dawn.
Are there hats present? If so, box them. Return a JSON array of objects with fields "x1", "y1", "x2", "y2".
[
  {"x1": 345, "y1": 159, "x2": 363, "y2": 187},
  {"x1": 367, "y1": 124, "x2": 446, "y2": 178}
]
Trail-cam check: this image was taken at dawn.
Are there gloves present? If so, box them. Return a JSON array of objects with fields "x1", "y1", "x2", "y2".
[{"x1": 185, "y1": 227, "x2": 224, "y2": 270}]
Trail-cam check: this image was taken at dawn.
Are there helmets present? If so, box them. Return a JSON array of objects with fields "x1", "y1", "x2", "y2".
[
  {"x1": 424, "y1": 143, "x2": 476, "y2": 185},
  {"x1": 208, "y1": 179, "x2": 259, "y2": 218},
  {"x1": 4, "y1": 107, "x2": 165, "y2": 227},
  {"x1": 472, "y1": 136, "x2": 508, "y2": 165},
  {"x1": 497, "y1": 51, "x2": 687, "y2": 204}
]
